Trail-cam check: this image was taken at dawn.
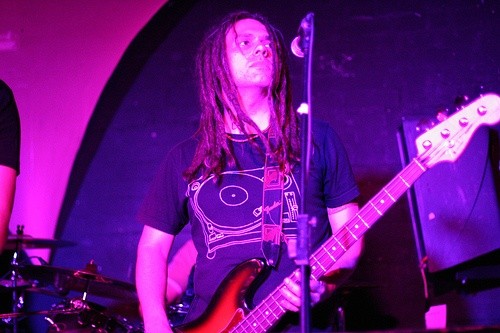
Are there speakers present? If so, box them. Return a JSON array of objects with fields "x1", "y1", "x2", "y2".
[{"x1": 396, "y1": 115, "x2": 500, "y2": 297}]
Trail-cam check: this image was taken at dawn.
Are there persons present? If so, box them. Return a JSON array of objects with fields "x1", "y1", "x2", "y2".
[
  {"x1": 134, "y1": 9, "x2": 365, "y2": 333},
  {"x1": 0, "y1": 78, "x2": 21, "y2": 252}
]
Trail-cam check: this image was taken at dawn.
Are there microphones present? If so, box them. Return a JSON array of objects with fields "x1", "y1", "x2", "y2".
[{"x1": 291, "y1": 13, "x2": 314, "y2": 57}]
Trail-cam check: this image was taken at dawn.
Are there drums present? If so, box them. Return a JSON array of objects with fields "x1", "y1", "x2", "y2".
[{"x1": 45, "y1": 296, "x2": 133, "y2": 333}]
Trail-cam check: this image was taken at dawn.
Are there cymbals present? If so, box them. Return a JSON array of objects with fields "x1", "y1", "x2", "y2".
[
  {"x1": 26, "y1": 264, "x2": 136, "y2": 293},
  {"x1": 0, "y1": 279, "x2": 30, "y2": 287},
  {"x1": 6, "y1": 234, "x2": 80, "y2": 249}
]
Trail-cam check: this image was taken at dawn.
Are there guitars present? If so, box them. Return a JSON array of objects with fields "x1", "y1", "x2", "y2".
[{"x1": 171, "y1": 82, "x2": 500, "y2": 333}]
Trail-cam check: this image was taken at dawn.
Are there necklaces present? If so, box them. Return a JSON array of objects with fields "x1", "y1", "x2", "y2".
[{"x1": 223, "y1": 123, "x2": 273, "y2": 144}]
